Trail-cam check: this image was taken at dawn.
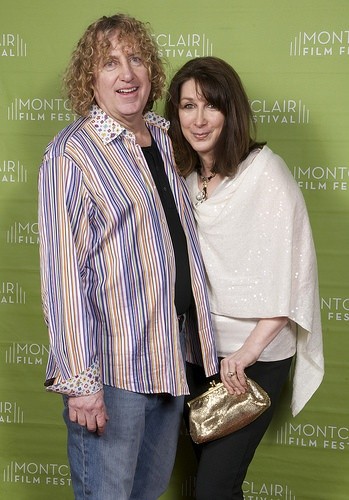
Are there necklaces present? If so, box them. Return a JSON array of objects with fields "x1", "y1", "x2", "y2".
[{"x1": 193, "y1": 170, "x2": 219, "y2": 205}]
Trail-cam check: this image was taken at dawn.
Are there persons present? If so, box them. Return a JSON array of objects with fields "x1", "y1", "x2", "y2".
[
  {"x1": 36, "y1": 15, "x2": 217, "y2": 500},
  {"x1": 161, "y1": 52, "x2": 326, "y2": 499}
]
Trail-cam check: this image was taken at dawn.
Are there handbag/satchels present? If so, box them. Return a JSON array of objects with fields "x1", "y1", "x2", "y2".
[{"x1": 188, "y1": 373, "x2": 271, "y2": 444}]
